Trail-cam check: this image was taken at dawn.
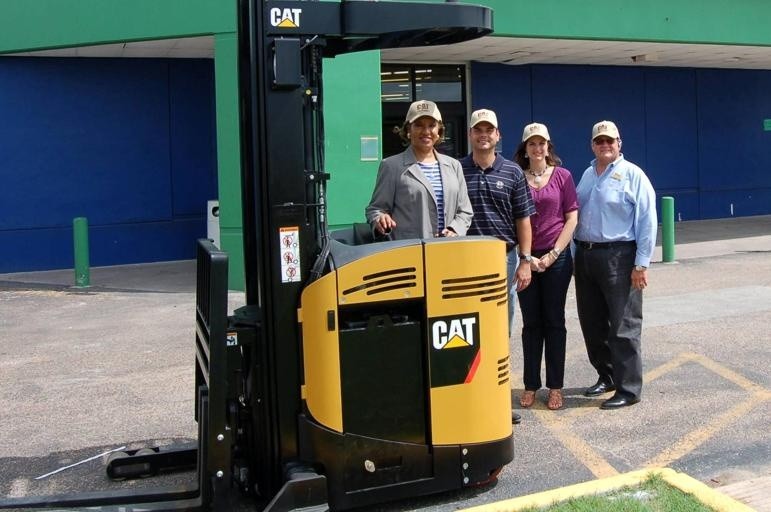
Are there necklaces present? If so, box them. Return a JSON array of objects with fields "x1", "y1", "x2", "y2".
[{"x1": 529, "y1": 164, "x2": 550, "y2": 183}]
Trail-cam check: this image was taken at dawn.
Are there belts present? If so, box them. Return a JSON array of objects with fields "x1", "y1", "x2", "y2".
[{"x1": 575, "y1": 239, "x2": 635, "y2": 250}]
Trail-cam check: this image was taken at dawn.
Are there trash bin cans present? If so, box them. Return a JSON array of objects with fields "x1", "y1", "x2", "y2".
[{"x1": 207, "y1": 200, "x2": 220, "y2": 250}]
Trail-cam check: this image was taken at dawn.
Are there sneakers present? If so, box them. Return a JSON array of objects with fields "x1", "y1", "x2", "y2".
[
  {"x1": 549, "y1": 389, "x2": 563, "y2": 409},
  {"x1": 521, "y1": 390, "x2": 536, "y2": 407}
]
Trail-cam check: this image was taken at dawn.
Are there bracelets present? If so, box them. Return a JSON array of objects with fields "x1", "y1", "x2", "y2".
[{"x1": 550, "y1": 248, "x2": 563, "y2": 261}]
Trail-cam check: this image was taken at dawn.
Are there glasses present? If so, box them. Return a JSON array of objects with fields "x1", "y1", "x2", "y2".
[{"x1": 595, "y1": 138, "x2": 616, "y2": 144}]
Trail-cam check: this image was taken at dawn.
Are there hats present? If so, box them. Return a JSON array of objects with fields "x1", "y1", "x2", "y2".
[
  {"x1": 592, "y1": 121, "x2": 621, "y2": 144},
  {"x1": 406, "y1": 100, "x2": 443, "y2": 124},
  {"x1": 523, "y1": 122, "x2": 551, "y2": 142},
  {"x1": 471, "y1": 109, "x2": 498, "y2": 129}
]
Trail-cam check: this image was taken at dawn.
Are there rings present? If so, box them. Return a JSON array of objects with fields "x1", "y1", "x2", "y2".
[{"x1": 526, "y1": 282, "x2": 529, "y2": 286}]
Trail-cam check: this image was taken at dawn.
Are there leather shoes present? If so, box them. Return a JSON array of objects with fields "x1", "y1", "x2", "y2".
[
  {"x1": 602, "y1": 395, "x2": 641, "y2": 408},
  {"x1": 587, "y1": 379, "x2": 617, "y2": 396},
  {"x1": 513, "y1": 411, "x2": 522, "y2": 423}
]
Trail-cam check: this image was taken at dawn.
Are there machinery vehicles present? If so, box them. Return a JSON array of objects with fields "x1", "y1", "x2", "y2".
[{"x1": 0, "y1": 0, "x2": 515, "y2": 512}]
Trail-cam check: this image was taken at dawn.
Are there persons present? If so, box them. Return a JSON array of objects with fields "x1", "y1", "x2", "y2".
[
  {"x1": 456, "y1": 108, "x2": 538, "y2": 423},
  {"x1": 511, "y1": 122, "x2": 580, "y2": 411},
  {"x1": 364, "y1": 101, "x2": 474, "y2": 240},
  {"x1": 576, "y1": 120, "x2": 660, "y2": 410}
]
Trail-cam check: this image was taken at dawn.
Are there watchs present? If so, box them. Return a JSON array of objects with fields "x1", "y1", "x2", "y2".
[
  {"x1": 633, "y1": 265, "x2": 647, "y2": 271},
  {"x1": 521, "y1": 254, "x2": 532, "y2": 263}
]
